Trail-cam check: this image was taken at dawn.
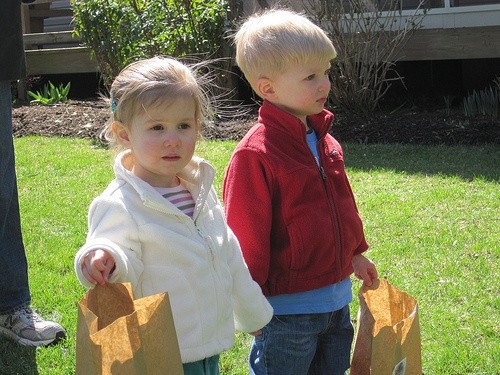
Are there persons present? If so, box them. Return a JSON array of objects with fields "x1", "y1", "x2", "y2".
[
  {"x1": 221, "y1": 8, "x2": 378, "y2": 375},
  {"x1": 74, "y1": 56, "x2": 275, "y2": 375},
  {"x1": 0, "y1": 0, "x2": 66, "y2": 348}
]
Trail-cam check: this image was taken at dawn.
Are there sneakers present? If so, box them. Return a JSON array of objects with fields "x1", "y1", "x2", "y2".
[{"x1": 0, "y1": 305, "x2": 66, "y2": 348}]
